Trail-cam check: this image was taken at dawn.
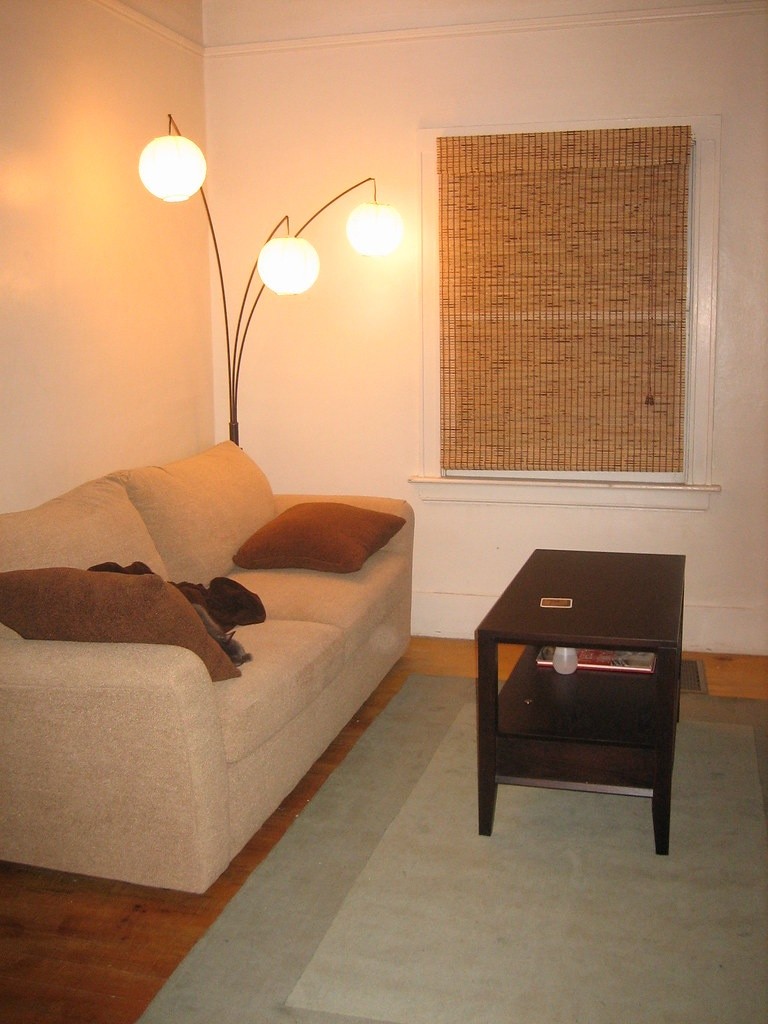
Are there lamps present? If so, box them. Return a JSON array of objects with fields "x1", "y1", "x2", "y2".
[{"x1": 137, "y1": 113, "x2": 403, "y2": 449}]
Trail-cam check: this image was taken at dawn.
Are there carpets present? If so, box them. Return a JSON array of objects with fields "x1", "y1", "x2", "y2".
[{"x1": 137, "y1": 671, "x2": 768, "y2": 1024}]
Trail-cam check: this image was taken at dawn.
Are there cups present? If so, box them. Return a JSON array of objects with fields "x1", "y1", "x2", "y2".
[{"x1": 553, "y1": 647, "x2": 578, "y2": 674}]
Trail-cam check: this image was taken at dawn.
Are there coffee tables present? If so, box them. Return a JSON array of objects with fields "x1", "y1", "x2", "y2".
[{"x1": 474, "y1": 550, "x2": 687, "y2": 858}]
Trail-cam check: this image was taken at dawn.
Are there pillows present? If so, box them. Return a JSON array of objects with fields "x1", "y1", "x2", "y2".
[
  {"x1": 0, "y1": 567, "x2": 242, "y2": 684},
  {"x1": 231, "y1": 502, "x2": 406, "y2": 574}
]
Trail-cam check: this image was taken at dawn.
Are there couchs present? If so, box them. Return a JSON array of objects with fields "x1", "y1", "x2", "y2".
[{"x1": 0, "y1": 442, "x2": 415, "y2": 895}]
